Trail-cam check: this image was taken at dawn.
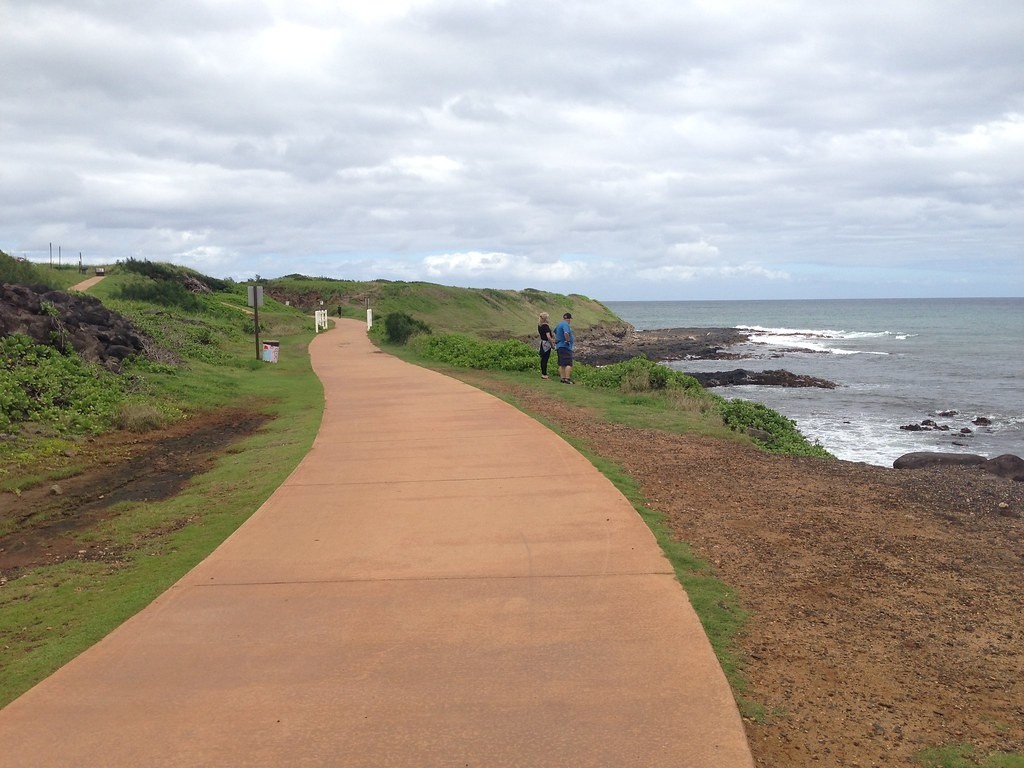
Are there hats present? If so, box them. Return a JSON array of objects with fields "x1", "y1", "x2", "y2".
[{"x1": 563, "y1": 313, "x2": 573, "y2": 319}]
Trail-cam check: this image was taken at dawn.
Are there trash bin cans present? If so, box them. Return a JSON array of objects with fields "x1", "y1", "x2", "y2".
[{"x1": 262, "y1": 340, "x2": 280, "y2": 364}]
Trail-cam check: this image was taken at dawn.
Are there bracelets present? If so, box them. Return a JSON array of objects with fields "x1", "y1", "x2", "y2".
[{"x1": 567, "y1": 340, "x2": 570, "y2": 342}]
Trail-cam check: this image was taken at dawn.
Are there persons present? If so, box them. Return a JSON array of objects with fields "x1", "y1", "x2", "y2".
[
  {"x1": 538, "y1": 312, "x2": 555, "y2": 379},
  {"x1": 553, "y1": 313, "x2": 576, "y2": 385},
  {"x1": 337, "y1": 305, "x2": 341, "y2": 318}
]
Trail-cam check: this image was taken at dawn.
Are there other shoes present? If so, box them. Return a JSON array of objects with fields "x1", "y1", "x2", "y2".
[
  {"x1": 561, "y1": 378, "x2": 575, "y2": 384},
  {"x1": 541, "y1": 375, "x2": 549, "y2": 379}
]
